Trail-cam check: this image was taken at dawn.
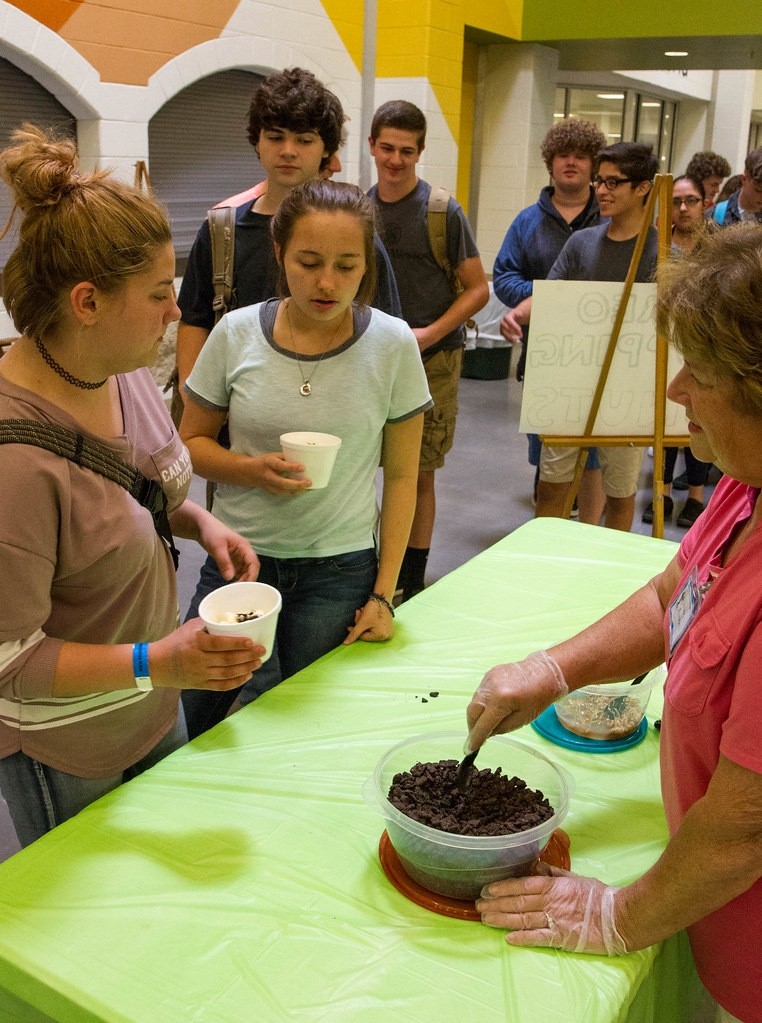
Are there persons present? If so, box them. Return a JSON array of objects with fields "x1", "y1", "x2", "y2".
[
  {"x1": 178, "y1": 181, "x2": 434, "y2": 745},
  {"x1": 196, "y1": 110, "x2": 350, "y2": 208},
  {"x1": 178, "y1": 66, "x2": 403, "y2": 716},
  {"x1": 492, "y1": 116, "x2": 761, "y2": 534},
  {"x1": 467, "y1": 220, "x2": 762, "y2": 1023},
  {"x1": 0, "y1": 122, "x2": 261, "y2": 853},
  {"x1": 357, "y1": 100, "x2": 490, "y2": 604}
]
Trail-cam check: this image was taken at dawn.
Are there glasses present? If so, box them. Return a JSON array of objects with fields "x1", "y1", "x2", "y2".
[
  {"x1": 591, "y1": 173, "x2": 651, "y2": 190},
  {"x1": 672, "y1": 197, "x2": 704, "y2": 208}
]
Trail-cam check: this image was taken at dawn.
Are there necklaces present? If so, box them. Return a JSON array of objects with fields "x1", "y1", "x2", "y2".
[
  {"x1": 25, "y1": 326, "x2": 111, "y2": 390},
  {"x1": 286, "y1": 297, "x2": 346, "y2": 396}
]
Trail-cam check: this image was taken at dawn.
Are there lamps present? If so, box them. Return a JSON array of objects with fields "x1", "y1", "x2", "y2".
[{"x1": 663, "y1": 38, "x2": 689, "y2": 57}]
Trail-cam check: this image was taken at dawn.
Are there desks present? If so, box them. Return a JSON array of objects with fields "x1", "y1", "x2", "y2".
[{"x1": 0, "y1": 516, "x2": 739, "y2": 1023}]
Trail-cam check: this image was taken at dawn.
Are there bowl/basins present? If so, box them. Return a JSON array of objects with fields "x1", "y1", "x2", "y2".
[
  {"x1": 198, "y1": 582, "x2": 282, "y2": 666},
  {"x1": 552, "y1": 663, "x2": 664, "y2": 740},
  {"x1": 363, "y1": 729, "x2": 575, "y2": 899},
  {"x1": 279, "y1": 431, "x2": 342, "y2": 489}
]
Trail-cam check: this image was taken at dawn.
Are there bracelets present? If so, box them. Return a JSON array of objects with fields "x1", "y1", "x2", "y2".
[
  {"x1": 133, "y1": 642, "x2": 154, "y2": 693},
  {"x1": 368, "y1": 593, "x2": 394, "y2": 617}
]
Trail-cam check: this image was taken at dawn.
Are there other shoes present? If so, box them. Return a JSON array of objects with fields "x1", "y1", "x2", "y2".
[
  {"x1": 531, "y1": 485, "x2": 537, "y2": 507},
  {"x1": 648, "y1": 446, "x2": 653, "y2": 457},
  {"x1": 571, "y1": 498, "x2": 579, "y2": 516},
  {"x1": 673, "y1": 468, "x2": 690, "y2": 490}
]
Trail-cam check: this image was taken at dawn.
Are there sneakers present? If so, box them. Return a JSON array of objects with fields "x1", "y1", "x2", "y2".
[
  {"x1": 677, "y1": 498, "x2": 705, "y2": 526},
  {"x1": 642, "y1": 495, "x2": 674, "y2": 522}
]
